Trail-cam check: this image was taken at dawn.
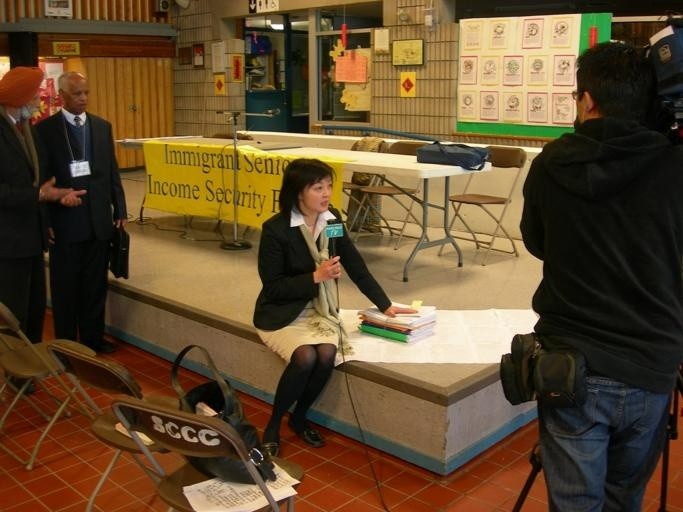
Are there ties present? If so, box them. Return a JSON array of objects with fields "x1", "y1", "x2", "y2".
[{"x1": 73, "y1": 117, "x2": 81, "y2": 128}]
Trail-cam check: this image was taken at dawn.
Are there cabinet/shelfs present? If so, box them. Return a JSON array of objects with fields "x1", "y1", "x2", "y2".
[{"x1": 242, "y1": 19, "x2": 289, "y2": 133}]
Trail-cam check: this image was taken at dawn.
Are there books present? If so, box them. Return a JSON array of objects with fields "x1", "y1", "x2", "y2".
[{"x1": 356, "y1": 301, "x2": 441, "y2": 343}]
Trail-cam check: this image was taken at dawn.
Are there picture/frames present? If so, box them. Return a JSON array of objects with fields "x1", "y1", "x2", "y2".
[{"x1": 392, "y1": 40, "x2": 424, "y2": 66}]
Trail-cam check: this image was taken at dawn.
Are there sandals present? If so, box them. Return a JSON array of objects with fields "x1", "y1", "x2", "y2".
[
  {"x1": 262, "y1": 428, "x2": 279, "y2": 457},
  {"x1": 291, "y1": 424, "x2": 325, "y2": 447}
]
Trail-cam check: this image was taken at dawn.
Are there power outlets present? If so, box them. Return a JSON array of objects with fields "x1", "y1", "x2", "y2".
[{"x1": 424, "y1": 9, "x2": 438, "y2": 32}]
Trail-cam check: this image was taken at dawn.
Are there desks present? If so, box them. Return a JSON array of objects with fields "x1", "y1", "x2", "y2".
[{"x1": 112, "y1": 135, "x2": 492, "y2": 283}]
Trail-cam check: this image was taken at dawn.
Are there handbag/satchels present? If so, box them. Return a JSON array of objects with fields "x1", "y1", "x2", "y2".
[{"x1": 108, "y1": 220, "x2": 130, "y2": 281}]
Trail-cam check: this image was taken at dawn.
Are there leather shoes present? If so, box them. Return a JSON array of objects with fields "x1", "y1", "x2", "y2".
[
  {"x1": 5, "y1": 371, "x2": 35, "y2": 394},
  {"x1": 79, "y1": 336, "x2": 114, "y2": 355}
]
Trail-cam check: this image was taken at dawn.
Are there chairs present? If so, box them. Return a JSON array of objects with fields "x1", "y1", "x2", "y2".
[
  {"x1": 360, "y1": 140, "x2": 430, "y2": 251},
  {"x1": 439, "y1": 145, "x2": 527, "y2": 267},
  {"x1": 111, "y1": 397, "x2": 305, "y2": 512},
  {"x1": 336, "y1": 136, "x2": 393, "y2": 243},
  {"x1": 46, "y1": 342, "x2": 180, "y2": 512},
  {"x1": 0, "y1": 304, "x2": 103, "y2": 470}
]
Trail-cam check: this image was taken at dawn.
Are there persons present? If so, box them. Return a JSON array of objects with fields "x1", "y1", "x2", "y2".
[
  {"x1": 520, "y1": 42, "x2": 683, "y2": 512},
  {"x1": 30, "y1": 71, "x2": 128, "y2": 367},
  {"x1": 252, "y1": 158, "x2": 421, "y2": 459},
  {"x1": 0, "y1": 65, "x2": 77, "y2": 393}
]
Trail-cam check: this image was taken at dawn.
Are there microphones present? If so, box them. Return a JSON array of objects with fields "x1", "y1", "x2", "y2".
[{"x1": 327, "y1": 218, "x2": 340, "y2": 281}]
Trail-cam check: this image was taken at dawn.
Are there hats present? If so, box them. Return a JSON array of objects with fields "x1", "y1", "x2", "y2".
[{"x1": 0, "y1": 66, "x2": 44, "y2": 108}]
